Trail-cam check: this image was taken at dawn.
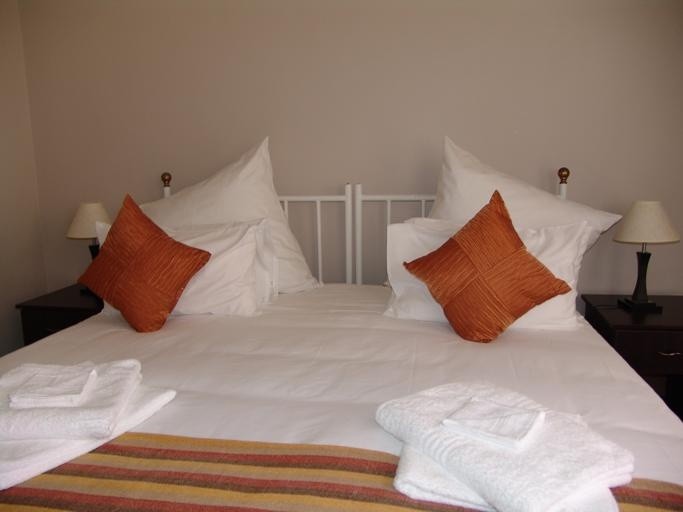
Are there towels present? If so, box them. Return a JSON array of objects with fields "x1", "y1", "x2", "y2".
[
  {"x1": 443, "y1": 396, "x2": 544, "y2": 450},
  {"x1": 8, "y1": 368, "x2": 95, "y2": 410}
]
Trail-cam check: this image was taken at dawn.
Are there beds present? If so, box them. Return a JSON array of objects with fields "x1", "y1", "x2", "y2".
[{"x1": 1, "y1": 167, "x2": 683, "y2": 511}]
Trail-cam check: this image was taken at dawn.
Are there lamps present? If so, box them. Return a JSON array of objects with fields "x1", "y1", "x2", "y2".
[
  {"x1": 66, "y1": 202, "x2": 114, "y2": 295},
  {"x1": 610, "y1": 200, "x2": 679, "y2": 313}
]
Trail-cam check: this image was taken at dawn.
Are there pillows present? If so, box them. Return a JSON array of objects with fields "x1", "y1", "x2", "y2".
[
  {"x1": 74, "y1": 192, "x2": 211, "y2": 334},
  {"x1": 424, "y1": 136, "x2": 624, "y2": 251},
  {"x1": 383, "y1": 218, "x2": 592, "y2": 330},
  {"x1": 94, "y1": 220, "x2": 277, "y2": 317},
  {"x1": 137, "y1": 136, "x2": 326, "y2": 297},
  {"x1": 403, "y1": 189, "x2": 571, "y2": 344}
]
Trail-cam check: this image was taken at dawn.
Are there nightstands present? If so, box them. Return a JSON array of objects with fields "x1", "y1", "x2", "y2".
[
  {"x1": 14, "y1": 283, "x2": 104, "y2": 350},
  {"x1": 581, "y1": 293, "x2": 682, "y2": 422}
]
